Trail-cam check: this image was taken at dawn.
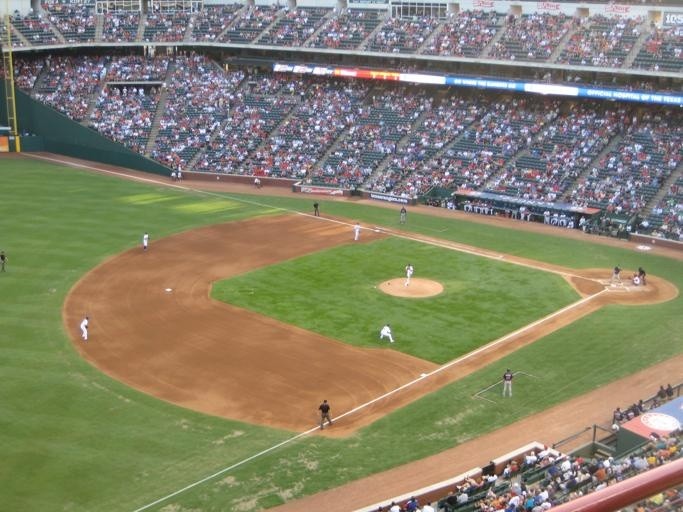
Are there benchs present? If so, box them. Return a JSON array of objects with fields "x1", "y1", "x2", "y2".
[
  {"x1": 439, "y1": 439, "x2": 682, "y2": 512},
  {"x1": 1, "y1": 0, "x2": 681, "y2": 251}
]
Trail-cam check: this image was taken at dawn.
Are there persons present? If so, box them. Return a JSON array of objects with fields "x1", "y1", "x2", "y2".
[
  {"x1": 613, "y1": 263, "x2": 623, "y2": 282},
  {"x1": 378, "y1": 427, "x2": 683, "y2": 512},
  {"x1": 633, "y1": 274, "x2": 641, "y2": 285},
  {"x1": 0, "y1": 250, "x2": 8, "y2": 272},
  {"x1": 405, "y1": 264, "x2": 413, "y2": 282},
  {"x1": 615, "y1": 384, "x2": 673, "y2": 423},
  {"x1": 1, "y1": 2, "x2": 682, "y2": 244},
  {"x1": 78, "y1": 317, "x2": 89, "y2": 340},
  {"x1": 638, "y1": 267, "x2": 646, "y2": 285},
  {"x1": 380, "y1": 324, "x2": 395, "y2": 344},
  {"x1": 503, "y1": 368, "x2": 512, "y2": 397},
  {"x1": 318, "y1": 399, "x2": 335, "y2": 430}
]
